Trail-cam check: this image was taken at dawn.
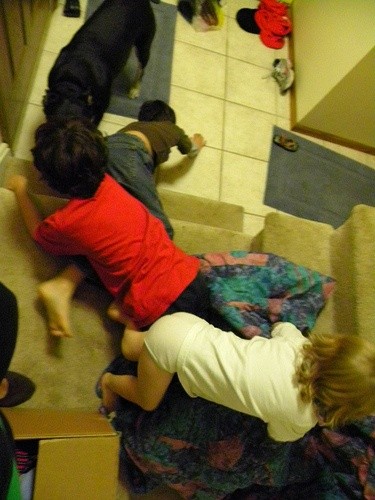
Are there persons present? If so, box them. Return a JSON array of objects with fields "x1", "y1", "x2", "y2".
[
  {"x1": 0, "y1": 282, "x2": 37, "y2": 407},
  {"x1": 7, "y1": 120, "x2": 246, "y2": 360},
  {"x1": 38, "y1": 99, "x2": 205, "y2": 338},
  {"x1": 101, "y1": 302, "x2": 375, "y2": 441}
]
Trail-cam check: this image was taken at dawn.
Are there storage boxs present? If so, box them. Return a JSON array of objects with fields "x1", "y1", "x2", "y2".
[{"x1": 0, "y1": 407, "x2": 119, "y2": 500}]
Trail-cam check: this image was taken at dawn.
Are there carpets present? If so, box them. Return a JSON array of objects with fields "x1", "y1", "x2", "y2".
[
  {"x1": 263, "y1": 125, "x2": 375, "y2": 228},
  {"x1": 84, "y1": 0, "x2": 176, "y2": 119}
]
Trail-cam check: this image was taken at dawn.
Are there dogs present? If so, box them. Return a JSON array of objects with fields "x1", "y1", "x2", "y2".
[{"x1": 41, "y1": 0, "x2": 157, "y2": 129}]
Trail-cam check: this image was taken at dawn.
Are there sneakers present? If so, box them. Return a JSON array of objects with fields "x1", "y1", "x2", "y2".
[{"x1": 271, "y1": 58, "x2": 294, "y2": 92}]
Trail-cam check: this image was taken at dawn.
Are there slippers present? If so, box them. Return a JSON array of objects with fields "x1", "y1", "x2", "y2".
[{"x1": 273, "y1": 134, "x2": 299, "y2": 152}]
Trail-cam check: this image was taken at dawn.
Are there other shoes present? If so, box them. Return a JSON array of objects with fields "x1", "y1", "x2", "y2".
[{"x1": 0, "y1": 371, "x2": 35, "y2": 407}]
